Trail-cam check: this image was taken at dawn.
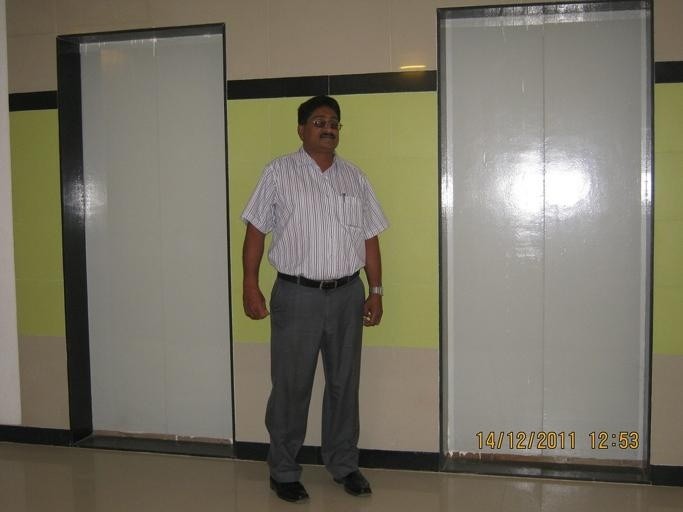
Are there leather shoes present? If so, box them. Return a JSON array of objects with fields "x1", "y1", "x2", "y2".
[
  {"x1": 270, "y1": 476, "x2": 309, "y2": 503},
  {"x1": 334, "y1": 470, "x2": 373, "y2": 498}
]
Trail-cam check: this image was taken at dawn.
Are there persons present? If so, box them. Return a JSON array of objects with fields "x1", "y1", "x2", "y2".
[{"x1": 240, "y1": 95, "x2": 384, "y2": 503}]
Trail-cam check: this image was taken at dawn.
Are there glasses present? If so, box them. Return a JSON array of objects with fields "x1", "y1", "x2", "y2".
[{"x1": 305, "y1": 119, "x2": 343, "y2": 129}]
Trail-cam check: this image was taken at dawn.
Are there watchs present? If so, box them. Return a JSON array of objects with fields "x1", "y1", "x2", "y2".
[{"x1": 368, "y1": 287, "x2": 384, "y2": 297}]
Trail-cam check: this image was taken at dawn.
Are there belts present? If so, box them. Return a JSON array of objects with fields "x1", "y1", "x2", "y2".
[{"x1": 277, "y1": 270, "x2": 359, "y2": 289}]
